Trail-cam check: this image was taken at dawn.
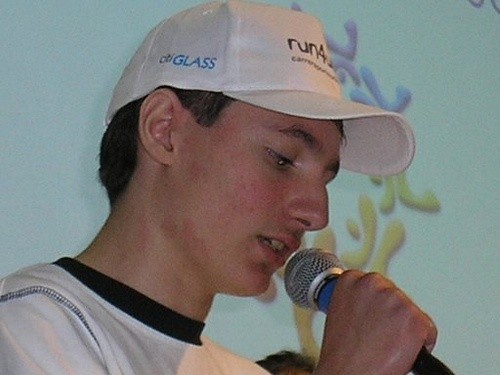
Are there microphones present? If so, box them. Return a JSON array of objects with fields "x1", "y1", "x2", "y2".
[{"x1": 283, "y1": 248, "x2": 455, "y2": 375}]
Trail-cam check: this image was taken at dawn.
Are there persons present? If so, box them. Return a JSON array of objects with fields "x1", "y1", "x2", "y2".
[{"x1": 0, "y1": 0, "x2": 437, "y2": 375}]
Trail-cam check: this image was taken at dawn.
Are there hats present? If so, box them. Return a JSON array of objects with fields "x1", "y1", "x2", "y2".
[{"x1": 105, "y1": 0, "x2": 416, "y2": 178}]
